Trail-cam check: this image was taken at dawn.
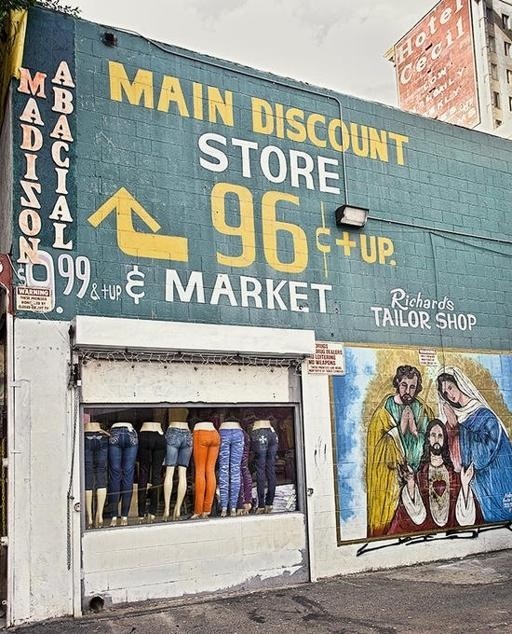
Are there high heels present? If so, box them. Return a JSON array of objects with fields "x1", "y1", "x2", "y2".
[
  {"x1": 187, "y1": 512, "x2": 211, "y2": 519},
  {"x1": 86, "y1": 511, "x2": 181, "y2": 529},
  {"x1": 221, "y1": 502, "x2": 273, "y2": 517}
]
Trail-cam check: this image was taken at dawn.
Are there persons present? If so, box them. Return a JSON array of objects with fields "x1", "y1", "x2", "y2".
[
  {"x1": 364, "y1": 364, "x2": 512, "y2": 537},
  {"x1": 84, "y1": 420, "x2": 279, "y2": 529}
]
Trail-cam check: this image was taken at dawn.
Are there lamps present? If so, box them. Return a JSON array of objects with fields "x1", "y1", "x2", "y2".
[{"x1": 335, "y1": 205, "x2": 369, "y2": 229}]
